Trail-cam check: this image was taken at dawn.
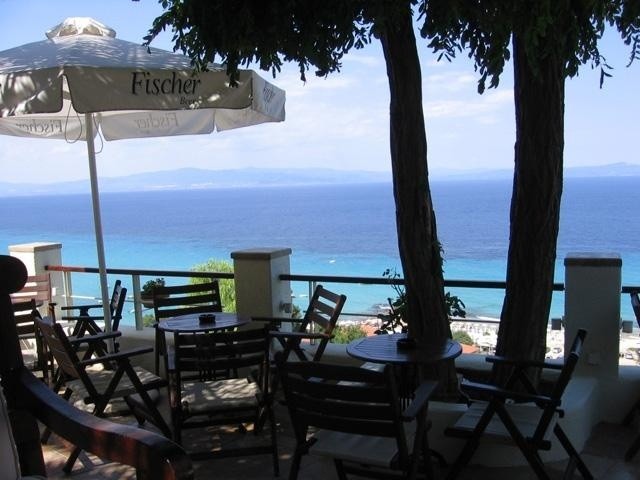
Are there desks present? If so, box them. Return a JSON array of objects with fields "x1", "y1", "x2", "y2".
[
  {"x1": 158, "y1": 312, "x2": 253, "y2": 380},
  {"x1": 345, "y1": 333, "x2": 463, "y2": 379}
]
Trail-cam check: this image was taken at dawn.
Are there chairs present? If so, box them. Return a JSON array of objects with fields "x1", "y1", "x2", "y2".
[
  {"x1": 52, "y1": 279, "x2": 128, "y2": 394},
  {"x1": 10, "y1": 298, "x2": 55, "y2": 387},
  {"x1": 34, "y1": 316, "x2": 173, "y2": 475},
  {"x1": 267, "y1": 284, "x2": 346, "y2": 395},
  {"x1": 152, "y1": 280, "x2": 239, "y2": 383},
  {"x1": 442, "y1": 328, "x2": 595, "y2": 480},
  {"x1": 8, "y1": 272, "x2": 52, "y2": 310},
  {"x1": 0, "y1": 255, "x2": 196, "y2": 479},
  {"x1": 167, "y1": 322, "x2": 280, "y2": 478},
  {"x1": 273, "y1": 348, "x2": 441, "y2": 480}
]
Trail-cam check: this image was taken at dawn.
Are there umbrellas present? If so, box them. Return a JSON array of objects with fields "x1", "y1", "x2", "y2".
[{"x1": 0, "y1": 16, "x2": 285, "y2": 370}]
